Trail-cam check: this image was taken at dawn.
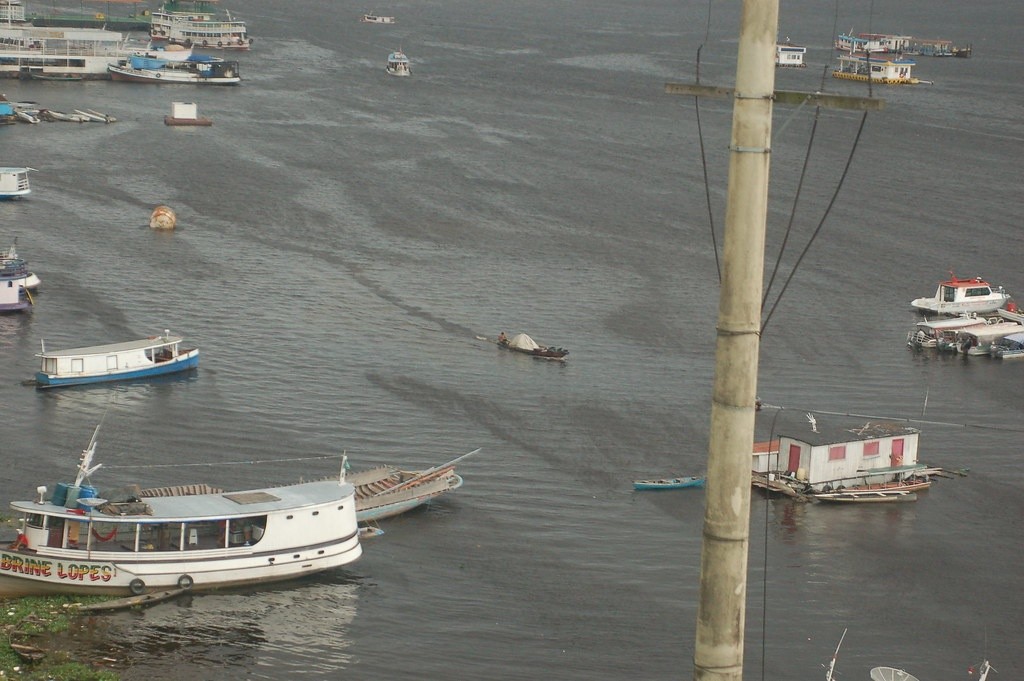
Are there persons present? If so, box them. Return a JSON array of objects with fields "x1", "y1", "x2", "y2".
[
  {"x1": 917, "y1": 326, "x2": 973, "y2": 350},
  {"x1": 9, "y1": 529, "x2": 28, "y2": 551},
  {"x1": 498, "y1": 333, "x2": 507, "y2": 343}
]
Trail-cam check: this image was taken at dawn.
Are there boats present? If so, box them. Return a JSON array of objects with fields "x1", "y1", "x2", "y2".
[
  {"x1": 951, "y1": 322, "x2": 1019, "y2": 356},
  {"x1": 0, "y1": 400, "x2": 362, "y2": 590},
  {"x1": 1, "y1": 0, "x2": 254, "y2": 87},
  {"x1": 361, "y1": 12, "x2": 397, "y2": 26},
  {"x1": 833, "y1": 49, "x2": 920, "y2": 86},
  {"x1": 119, "y1": 446, "x2": 485, "y2": 528},
  {"x1": 632, "y1": 474, "x2": 708, "y2": 496},
  {"x1": 817, "y1": 489, "x2": 917, "y2": 507},
  {"x1": 906, "y1": 274, "x2": 1011, "y2": 313},
  {"x1": 33, "y1": 325, "x2": 203, "y2": 388},
  {"x1": 0, "y1": 164, "x2": 33, "y2": 200},
  {"x1": 386, "y1": 43, "x2": 411, "y2": 77},
  {"x1": 902, "y1": 314, "x2": 984, "y2": 350},
  {"x1": 835, "y1": 29, "x2": 972, "y2": 59},
  {"x1": 497, "y1": 331, "x2": 570, "y2": 361},
  {"x1": 843, "y1": 464, "x2": 932, "y2": 493},
  {"x1": 990, "y1": 332, "x2": 1023, "y2": 360},
  {"x1": 776, "y1": 37, "x2": 808, "y2": 68},
  {"x1": 163, "y1": 101, "x2": 213, "y2": 127},
  {"x1": 1, "y1": 235, "x2": 40, "y2": 314},
  {"x1": 997, "y1": 305, "x2": 1024, "y2": 324}
]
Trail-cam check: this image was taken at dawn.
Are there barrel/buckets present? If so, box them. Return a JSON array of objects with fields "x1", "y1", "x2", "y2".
[
  {"x1": 53, "y1": 483, "x2": 98, "y2": 513},
  {"x1": 1007, "y1": 303, "x2": 1015, "y2": 312},
  {"x1": 797, "y1": 468, "x2": 805, "y2": 480}
]
